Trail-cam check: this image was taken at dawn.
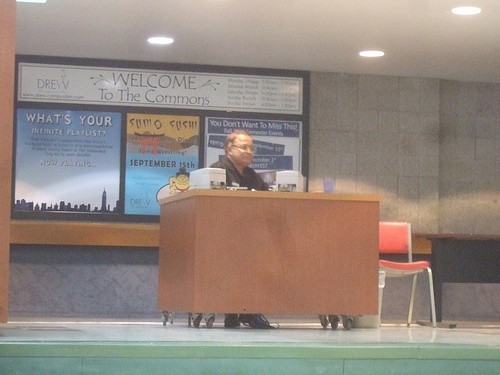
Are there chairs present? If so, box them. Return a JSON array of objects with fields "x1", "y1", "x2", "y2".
[{"x1": 379, "y1": 222, "x2": 437, "y2": 327}]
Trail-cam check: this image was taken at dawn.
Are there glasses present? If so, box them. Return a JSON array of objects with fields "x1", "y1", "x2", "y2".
[{"x1": 232, "y1": 145, "x2": 256, "y2": 153}]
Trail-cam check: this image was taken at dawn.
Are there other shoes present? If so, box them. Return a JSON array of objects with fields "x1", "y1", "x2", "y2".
[
  {"x1": 224, "y1": 313, "x2": 240, "y2": 329},
  {"x1": 239, "y1": 314, "x2": 268, "y2": 328}
]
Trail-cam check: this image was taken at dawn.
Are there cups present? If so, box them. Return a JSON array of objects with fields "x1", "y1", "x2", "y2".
[{"x1": 323, "y1": 176, "x2": 336, "y2": 194}]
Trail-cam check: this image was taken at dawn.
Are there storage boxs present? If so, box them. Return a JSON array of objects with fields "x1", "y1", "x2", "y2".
[
  {"x1": 189, "y1": 168, "x2": 226, "y2": 189},
  {"x1": 276, "y1": 171, "x2": 304, "y2": 192}
]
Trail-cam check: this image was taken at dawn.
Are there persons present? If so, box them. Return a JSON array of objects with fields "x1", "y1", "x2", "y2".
[{"x1": 209, "y1": 130, "x2": 276, "y2": 329}]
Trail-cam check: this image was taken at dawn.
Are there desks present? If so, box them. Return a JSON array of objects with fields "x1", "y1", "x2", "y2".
[{"x1": 159, "y1": 189, "x2": 379, "y2": 330}]
